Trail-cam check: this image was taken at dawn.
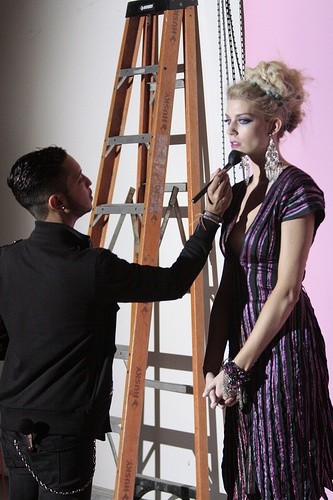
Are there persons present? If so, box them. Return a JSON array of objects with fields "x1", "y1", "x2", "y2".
[
  {"x1": 202, "y1": 60, "x2": 333, "y2": 500},
  {"x1": 0, "y1": 144, "x2": 233, "y2": 500}
]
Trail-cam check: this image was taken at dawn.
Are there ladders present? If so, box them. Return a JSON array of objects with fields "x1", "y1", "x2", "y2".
[{"x1": 67, "y1": 0, "x2": 212, "y2": 500}]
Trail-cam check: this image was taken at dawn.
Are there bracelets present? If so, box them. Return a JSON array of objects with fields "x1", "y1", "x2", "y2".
[
  {"x1": 191, "y1": 210, "x2": 224, "y2": 230},
  {"x1": 219, "y1": 357, "x2": 250, "y2": 410}
]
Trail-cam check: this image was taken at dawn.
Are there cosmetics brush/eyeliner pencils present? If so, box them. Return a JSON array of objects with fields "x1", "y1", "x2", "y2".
[{"x1": 191, "y1": 150, "x2": 243, "y2": 204}]
